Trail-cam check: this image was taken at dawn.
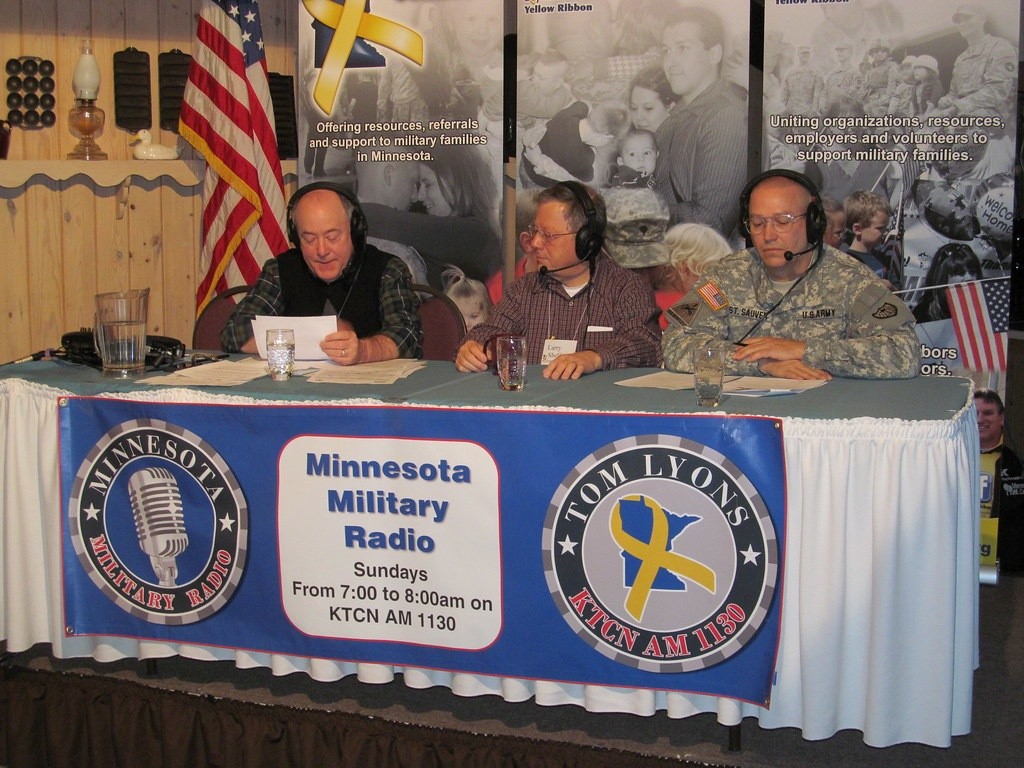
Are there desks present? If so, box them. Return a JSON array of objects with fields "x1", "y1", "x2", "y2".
[{"x1": 0, "y1": 360, "x2": 983, "y2": 748}]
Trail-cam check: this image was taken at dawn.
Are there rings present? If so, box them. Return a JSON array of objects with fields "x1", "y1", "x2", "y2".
[{"x1": 342, "y1": 350, "x2": 345, "y2": 357}]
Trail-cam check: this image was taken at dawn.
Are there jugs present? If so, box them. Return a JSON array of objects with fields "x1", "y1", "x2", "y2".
[{"x1": 93, "y1": 288, "x2": 152, "y2": 381}]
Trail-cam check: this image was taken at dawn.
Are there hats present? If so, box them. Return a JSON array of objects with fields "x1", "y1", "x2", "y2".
[
  {"x1": 868, "y1": 39, "x2": 892, "y2": 55},
  {"x1": 896, "y1": 55, "x2": 916, "y2": 71},
  {"x1": 797, "y1": 45, "x2": 812, "y2": 55},
  {"x1": 834, "y1": 42, "x2": 854, "y2": 53},
  {"x1": 911, "y1": 54, "x2": 939, "y2": 78},
  {"x1": 952, "y1": 2, "x2": 985, "y2": 22}
]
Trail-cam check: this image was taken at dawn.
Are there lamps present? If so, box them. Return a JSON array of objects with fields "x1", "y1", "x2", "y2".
[{"x1": 67, "y1": 40, "x2": 109, "y2": 159}]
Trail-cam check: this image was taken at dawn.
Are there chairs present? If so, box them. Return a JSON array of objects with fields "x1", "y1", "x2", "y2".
[
  {"x1": 412, "y1": 287, "x2": 472, "y2": 361},
  {"x1": 193, "y1": 285, "x2": 254, "y2": 351}
]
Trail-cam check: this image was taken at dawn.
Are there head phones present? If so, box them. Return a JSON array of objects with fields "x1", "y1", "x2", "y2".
[
  {"x1": 558, "y1": 180, "x2": 602, "y2": 261},
  {"x1": 287, "y1": 181, "x2": 368, "y2": 256},
  {"x1": 739, "y1": 169, "x2": 826, "y2": 244}
]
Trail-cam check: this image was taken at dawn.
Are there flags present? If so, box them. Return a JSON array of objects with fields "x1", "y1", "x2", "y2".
[
  {"x1": 945, "y1": 275, "x2": 1008, "y2": 371},
  {"x1": 880, "y1": 196, "x2": 904, "y2": 301},
  {"x1": 894, "y1": 128, "x2": 922, "y2": 196}
]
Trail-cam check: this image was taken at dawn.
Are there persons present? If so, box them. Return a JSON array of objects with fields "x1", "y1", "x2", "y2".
[
  {"x1": 661, "y1": 168, "x2": 920, "y2": 382},
  {"x1": 297, "y1": 0, "x2": 1023, "y2": 518},
  {"x1": 454, "y1": 181, "x2": 663, "y2": 380},
  {"x1": 221, "y1": 182, "x2": 423, "y2": 367},
  {"x1": 179, "y1": 0, "x2": 292, "y2": 321}
]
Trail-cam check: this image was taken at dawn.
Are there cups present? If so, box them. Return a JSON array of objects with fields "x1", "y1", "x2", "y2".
[
  {"x1": 483, "y1": 332, "x2": 523, "y2": 375},
  {"x1": 692, "y1": 348, "x2": 725, "y2": 408},
  {"x1": 265, "y1": 329, "x2": 296, "y2": 381},
  {"x1": 495, "y1": 336, "x2": 527, "y2": 391}
]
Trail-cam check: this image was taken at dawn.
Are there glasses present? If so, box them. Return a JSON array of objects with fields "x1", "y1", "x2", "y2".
[
  {"x1": 873, "y1": 48, "x2": 887, "y2": 53},
  {"x1": 743, "y1": 213, "x2": 808, "y2": 235},
  {"x1": 528, "y1": 223, "x2": 577, "y2": 241}
]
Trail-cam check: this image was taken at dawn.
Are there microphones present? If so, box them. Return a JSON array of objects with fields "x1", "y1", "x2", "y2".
[
  {"x1": 540, "y1": 241, "x2": 598, "y2": 275},
  {"x1": 784, "y1": 220, "x2": 826, "y2": 261},
  {"x1": 342, "y1": 244, "x2": 368, "y2": 276}
]
